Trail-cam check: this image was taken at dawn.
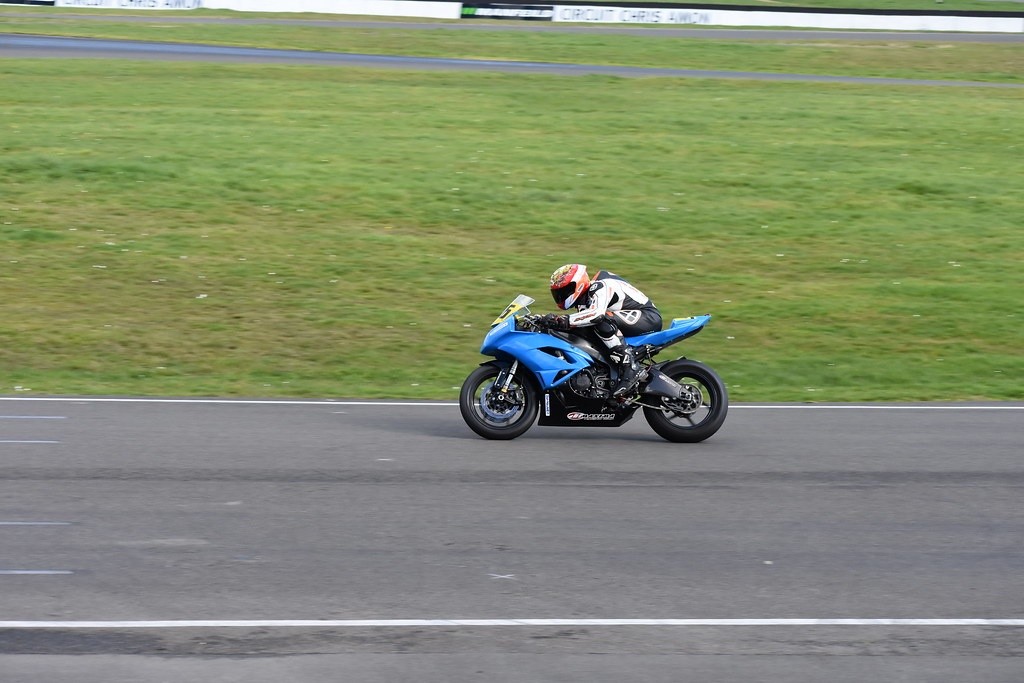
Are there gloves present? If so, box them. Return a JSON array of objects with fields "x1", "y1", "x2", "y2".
[{"x1": 537, "y1": 313, "x2": 569, "y2": 330}]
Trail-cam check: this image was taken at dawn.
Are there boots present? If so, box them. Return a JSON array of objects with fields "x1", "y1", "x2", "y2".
[{"x1": 610, "y1": 344, "x2": 648, "y2": 396}]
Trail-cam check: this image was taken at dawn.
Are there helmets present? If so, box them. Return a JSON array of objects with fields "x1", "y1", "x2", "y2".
[{"x1": 550, "y1": 263, "x2": 591, "y2": 310}]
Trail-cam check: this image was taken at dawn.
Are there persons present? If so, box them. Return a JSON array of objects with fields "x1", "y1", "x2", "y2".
[{"x1": 536, "y1": 264, "x2": 662, "y2": 397}]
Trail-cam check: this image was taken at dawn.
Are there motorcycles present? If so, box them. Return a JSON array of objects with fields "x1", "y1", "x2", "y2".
[{"x1": 458, "y1": 292, "x2": 730, "y2": 443}]
